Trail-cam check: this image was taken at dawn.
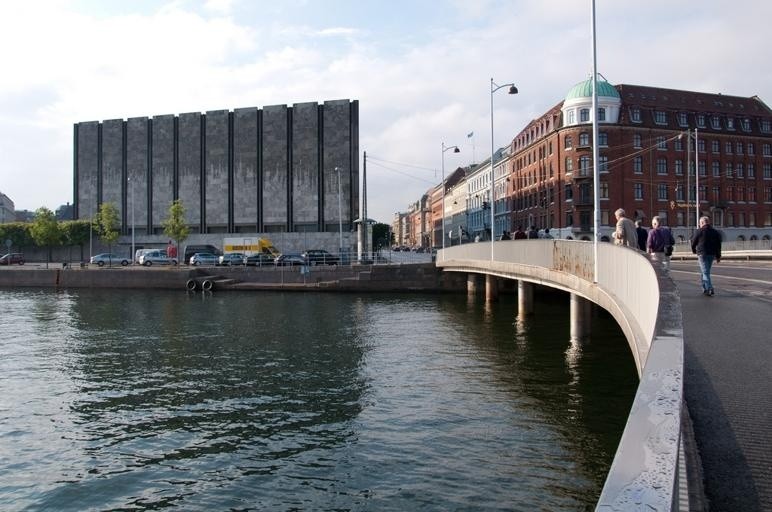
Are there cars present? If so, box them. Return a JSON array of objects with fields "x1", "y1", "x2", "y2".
[
  {"x1": 89, "y1": 249, "x2": 339, "y2": 266},
  {"x1": 1, "y1": 251, "x2": 24, "y2": 265},
  {"x1": 392, "y1": 242, "x2": 437, "y2": 254}
]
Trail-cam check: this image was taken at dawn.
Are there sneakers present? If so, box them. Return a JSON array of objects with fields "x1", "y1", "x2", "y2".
[{"x1": 704, "y1": 289, "x2": 714, "y2": 296}]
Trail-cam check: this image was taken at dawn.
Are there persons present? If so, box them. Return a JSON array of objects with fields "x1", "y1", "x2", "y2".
[
  {"x1": 612, "y1": 208, "x2": 675, "y2": 269},
  {"x1": 691, "y1": 216, "x2": 721, "y2": 296},
  {"x1": 474, "y1": 225, "x2": 553, "y2": 243}
]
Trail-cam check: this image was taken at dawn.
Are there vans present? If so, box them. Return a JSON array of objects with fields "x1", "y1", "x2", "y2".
[{"x1": 183, "y1": 243, "x2": 224, "y2": 264}]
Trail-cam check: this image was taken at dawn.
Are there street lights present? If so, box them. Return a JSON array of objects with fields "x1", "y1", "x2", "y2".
[
  {"x1": 334, "y1": 166, "x2": 341, "y2": 265},
  {"x1": 489, "y1": 75, "x2": 519, "y2": 260},
  {"x1": 440, "y1": 140, "x2": 461, "y2": 252},
  {"x1": 126, "y1": 175, "x2": 136, "y2": 263}
]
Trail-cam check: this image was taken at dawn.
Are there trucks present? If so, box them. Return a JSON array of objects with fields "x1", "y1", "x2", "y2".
[{"x1": 223, "y1": 237, "x2": 283, "y2": 258}]
